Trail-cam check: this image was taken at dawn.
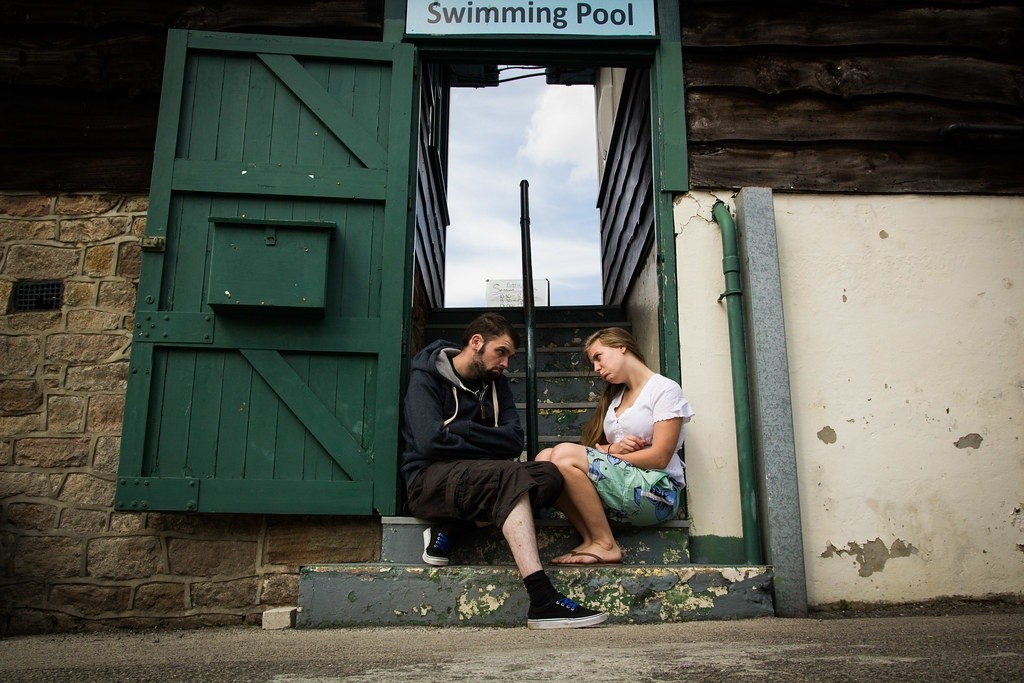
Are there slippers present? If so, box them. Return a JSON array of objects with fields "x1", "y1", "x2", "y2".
[{"x1": 548, "y1": 549, "x2": 624, "y2": 568}]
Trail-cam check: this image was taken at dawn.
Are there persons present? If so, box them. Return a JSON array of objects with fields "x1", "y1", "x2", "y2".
[
  {"x1": 534, "y1": 326, "x2": 694, "y2": 567},
  {"x1": 397, "y1": 310, "x2": 611, "y2": 630}
]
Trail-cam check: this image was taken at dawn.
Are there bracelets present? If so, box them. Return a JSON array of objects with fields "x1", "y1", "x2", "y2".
[{"x1": 607, "y1": 442, "x2": 613, "y2": 454}]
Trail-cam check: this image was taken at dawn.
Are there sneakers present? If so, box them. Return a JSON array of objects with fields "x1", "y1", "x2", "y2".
[
  {"x1": 527, "y1": 591, "x2": 610, "y2": 631},
  {"x1": 421, "y1": 526, "x2": 454, "y2": 567}
]
